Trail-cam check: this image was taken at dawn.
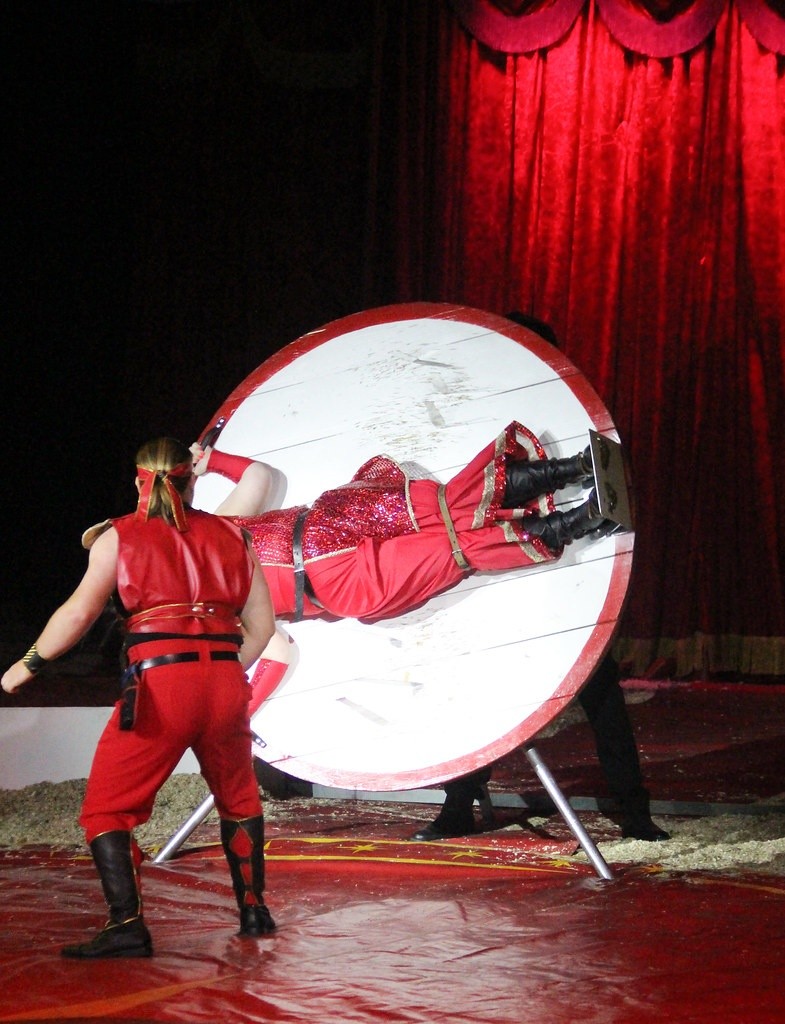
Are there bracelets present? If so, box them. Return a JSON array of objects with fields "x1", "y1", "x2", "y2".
[{"x1": 21, "y1": 642, "x2": 54, "y2": 677}]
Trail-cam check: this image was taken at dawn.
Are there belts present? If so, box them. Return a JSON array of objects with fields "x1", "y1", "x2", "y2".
[
  {"x1": 438, "y1": 483, "x2": 468, "y2": 570},
  {"x1": 293, "y1": 510, "x2": 325, "y2": 623},
  {"x1": 120, "y1": 651, "x2": 240, "y2": 730}
]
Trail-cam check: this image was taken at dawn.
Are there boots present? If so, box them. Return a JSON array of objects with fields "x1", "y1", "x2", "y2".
[
  {"x1": 517, "y1": 484, "x2": 626, "y2": 555},
  {"x1": 501, "y1": 440, "x2": 611, "y2": 510}
]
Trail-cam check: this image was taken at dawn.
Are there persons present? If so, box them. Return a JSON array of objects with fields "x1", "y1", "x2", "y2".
[
  {"x1": 409, "y1": 309, "x2": 671, "y2": 841},
  {"x1": 1, "y1": 437, "x2": 276, "y2": 961},
  {"x1": 189, "y1": 421, "x2": 622, "y2": 720}
]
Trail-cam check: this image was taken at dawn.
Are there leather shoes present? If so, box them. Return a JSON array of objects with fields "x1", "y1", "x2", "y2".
[
  {"x1": 60, "y1": 918, "x2": 153, "y2": 960},
  {"x1": 411, "y1": 784, "x2": 499, "y2": 842},
  {"x1": 239, "y1": 904, "x2": 275, "y2": 937},
  {"x1": 622, "y1": 813, "x2": 671, "y2": 841}
]
